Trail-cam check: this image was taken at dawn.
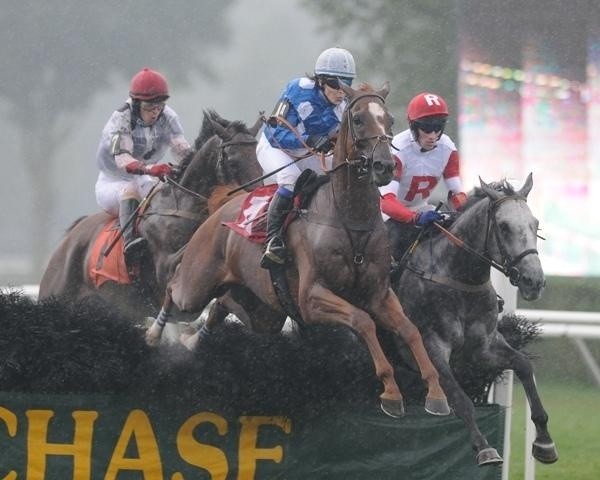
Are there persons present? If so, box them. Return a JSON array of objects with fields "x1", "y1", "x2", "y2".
[
  {"x1": 256, "y1": 48, "x2": 358, "y2": 270},
  {"x1": 95, "y1": 66, "x2": 195, "y2": 284},
  {"x1": 377, "y1": 94, "x2": 466, "y2": 275}
]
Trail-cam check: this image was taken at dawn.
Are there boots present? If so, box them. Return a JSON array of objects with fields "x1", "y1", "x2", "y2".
[
  {"x1": 262, "y1": 192, "x2": 291, "y2": 269},
  {"x1": 119, "y1": 197, "x2": 148, "y2": 256}
]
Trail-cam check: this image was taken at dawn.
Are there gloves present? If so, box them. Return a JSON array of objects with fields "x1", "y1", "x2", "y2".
[
  {"x1": 150, "y1": 162, "x2": 172, "y2": 177},
  {"x1": 417, "y1": 209, "x2": 442, "y2": 226},
  {"x1": 306, "y1": 133, "x2": 331, "y2": 153}
]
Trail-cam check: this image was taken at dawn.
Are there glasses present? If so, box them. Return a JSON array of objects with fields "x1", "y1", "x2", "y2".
[
  {"x1": 413, "y1": 122, "x2": 442, "y2": 133},
  {"x1": 322, "y1": 76, "x2": 352, "y2": 90},
  {"x1": 138, "y1": 101, "x2": 166, "y2": 111}
]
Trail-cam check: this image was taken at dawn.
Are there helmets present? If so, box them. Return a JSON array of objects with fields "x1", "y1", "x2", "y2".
[
  {"x1": 406, "y1": 92, "x2": 448, "y2": 119},
  {"x1": 128, "y1": 68, "x2": 171, "y2": 100},
  {"x1": 314, "y1": 47, "x2": 356, "y2": 78}
]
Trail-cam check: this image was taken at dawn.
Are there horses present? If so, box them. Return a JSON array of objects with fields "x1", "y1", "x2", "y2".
[
  {"x1": 144, "y1": 74, "x2": 452, "y2": 421},
  {"x1": 36, "y1": 106, "x2": 267, "y2": 306},
  {"x1": 385, "y1": 171, "x2": 560, "y2": 467}
]
